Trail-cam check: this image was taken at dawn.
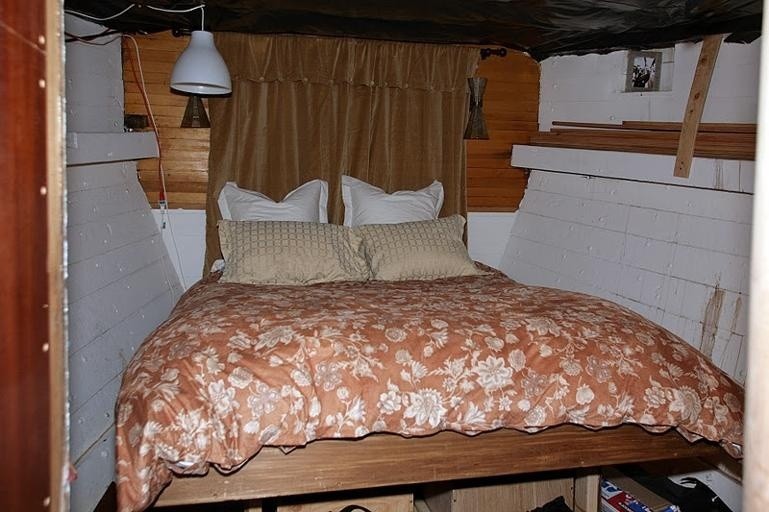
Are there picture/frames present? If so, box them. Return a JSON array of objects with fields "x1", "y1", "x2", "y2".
[{"x1": 625, "y1": 51, "x2": 662, "y2": 92}]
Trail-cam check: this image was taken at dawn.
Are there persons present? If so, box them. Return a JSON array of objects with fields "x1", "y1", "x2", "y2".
[{"x1": 631, "y1": 63, "x2": 656, "y2": 89}]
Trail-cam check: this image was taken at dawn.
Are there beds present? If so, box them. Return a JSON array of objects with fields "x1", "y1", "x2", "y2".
[{"x1": 115, "y1": 24, "x2": 744, "y2": 512}]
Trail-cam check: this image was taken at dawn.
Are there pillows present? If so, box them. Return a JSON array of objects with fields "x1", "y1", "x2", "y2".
[{"x1": 210, "y1": 175, "x2": 494, "y2": 286}]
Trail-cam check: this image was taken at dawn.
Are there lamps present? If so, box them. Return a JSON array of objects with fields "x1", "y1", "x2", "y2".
[
  {"x1": 169, "y1": 5, "x2": 232, "y2": 95},
  {"x1": 465, "y1": 77, "x2": 490, "y2": 140},
  {"x1": 179, "y1": 96, "x2": 211, "y2": 128}
]
